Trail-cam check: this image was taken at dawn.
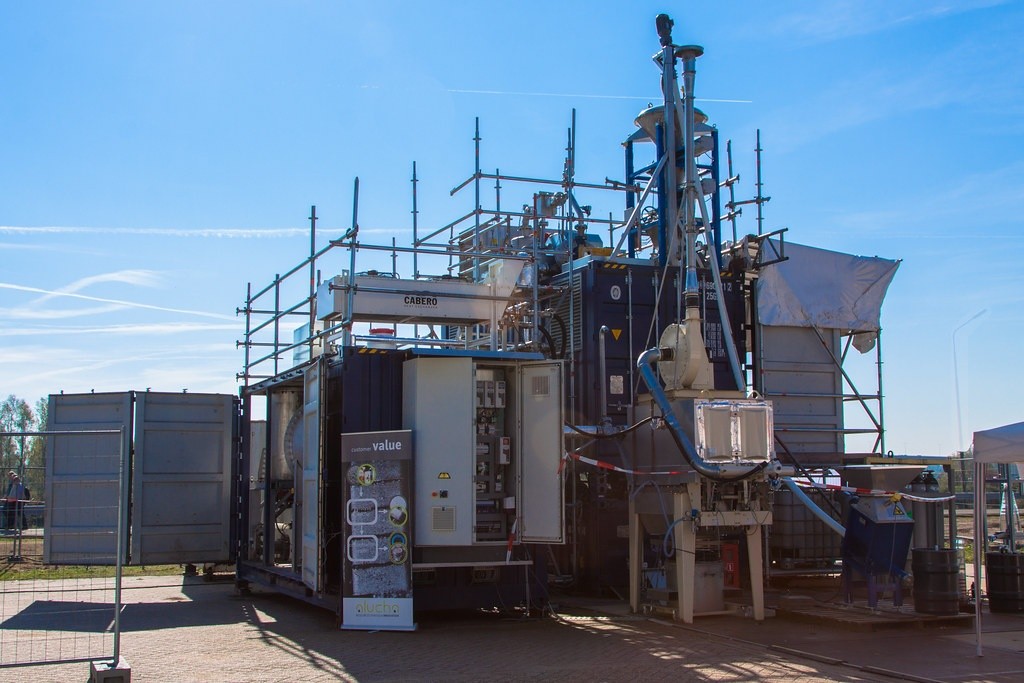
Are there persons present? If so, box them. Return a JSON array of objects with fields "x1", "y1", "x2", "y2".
[{"x1": 3, "y1": 471, "x2": 28, "y2": 530}]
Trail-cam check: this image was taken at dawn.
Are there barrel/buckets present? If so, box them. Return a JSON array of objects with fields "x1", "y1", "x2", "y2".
[
  {"x1": 910, "y1": 546, "x2": 961, "y2": 616},
  {"x1": 984, "y1": 550, "x2": 1024, "y2": 614}
]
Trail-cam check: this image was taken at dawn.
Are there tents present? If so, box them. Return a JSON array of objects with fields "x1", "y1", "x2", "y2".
[{"x1": 973, "y1": 422, "x2": 1024, "y2": 656}]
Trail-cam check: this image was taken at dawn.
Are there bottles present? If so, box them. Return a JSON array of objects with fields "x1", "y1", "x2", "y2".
[{"x1": 515, "y1": 251, "x2": 532, "y2": 286}]
